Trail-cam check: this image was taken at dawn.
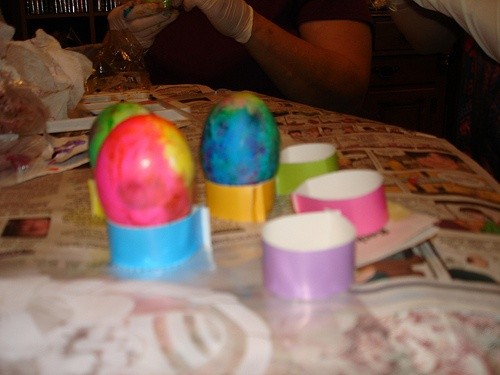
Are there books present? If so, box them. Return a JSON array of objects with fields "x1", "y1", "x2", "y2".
[{"x1": 23, "y1": 0, "x2": 122, "y2": 15}]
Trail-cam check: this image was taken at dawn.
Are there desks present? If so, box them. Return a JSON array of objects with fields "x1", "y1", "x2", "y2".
[{"x1": 0, "y1": 84, "x2": 500, "y2": 375}]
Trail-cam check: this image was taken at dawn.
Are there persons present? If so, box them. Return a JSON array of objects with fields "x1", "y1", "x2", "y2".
[{"x1": 108, "y1": 0, "x2": 375, "y2": 121}]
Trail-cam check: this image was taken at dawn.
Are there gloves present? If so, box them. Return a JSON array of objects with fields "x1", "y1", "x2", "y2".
[
  {"x1": 107, "y1": 3, "x2": 179, "y2": 50},
  {"x1": 183, "y1": 0, "x2": 254, "y2": 44}
]
